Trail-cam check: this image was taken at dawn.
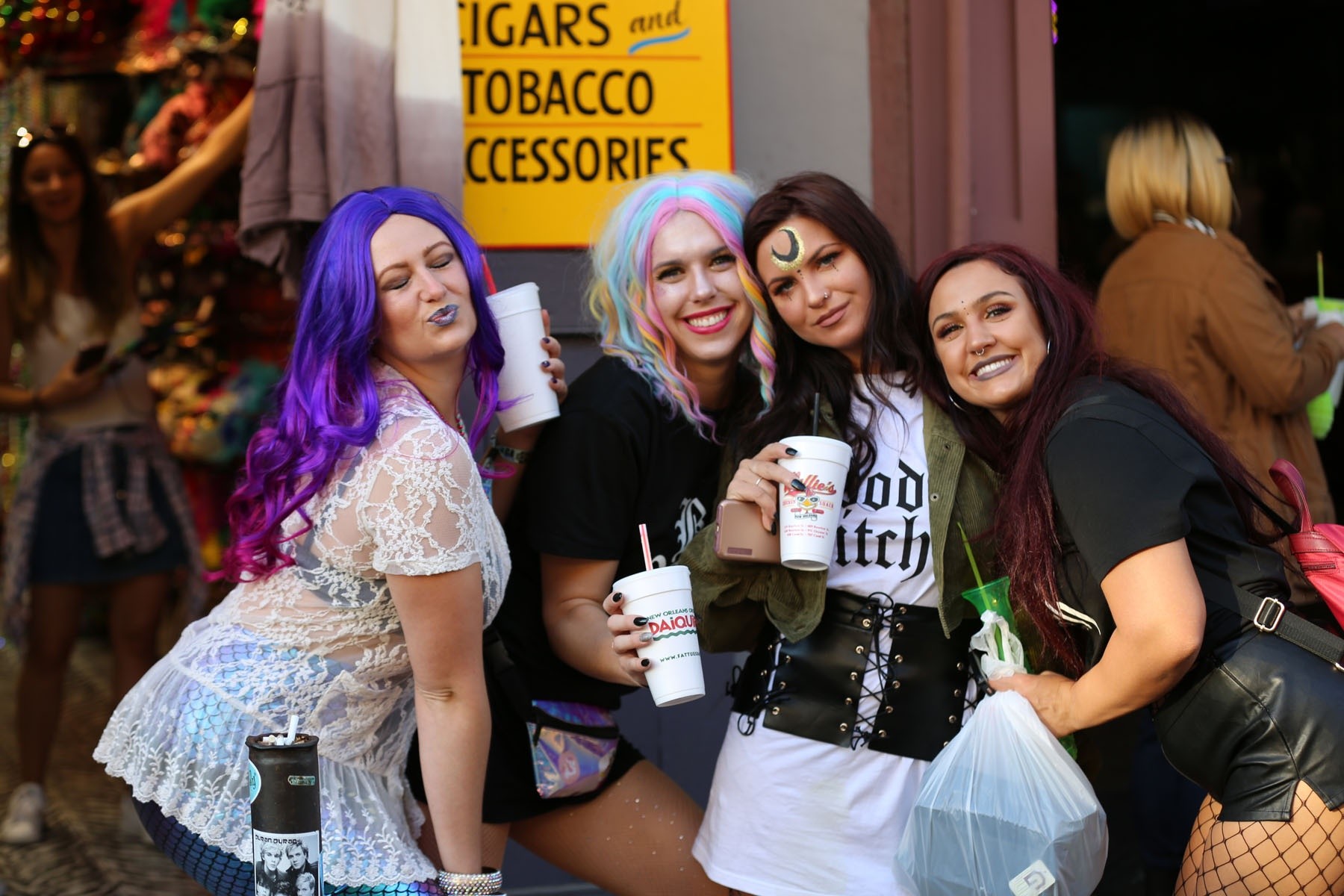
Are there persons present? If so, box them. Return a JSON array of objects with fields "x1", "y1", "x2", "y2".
[
  {"x1": 890, "y1": 241, "x2": 1344, "y2": 896},
  {"x1": 87, "y1": 187, "x2": 566, "y2": 896},
  {"x1": 1086, "y1": 112, "x2": 1344, "y2": 537},
  {"x1": 672, "y1": 170, "x2": 1004, "y2": 896},
  {"x1": 403, "y1": 170, "x2": 774, "y2": 896},
  {"x1": 254, "y1": 841, "x2": 316, "y2": 896},
  {"x1": 0, "y1": 82, "x2": 259, "y2": 850}
]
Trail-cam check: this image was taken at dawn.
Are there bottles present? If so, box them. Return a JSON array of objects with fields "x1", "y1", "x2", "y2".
[{"x1": 961, "y1": 576, "x2": 1078, "y2": 762}]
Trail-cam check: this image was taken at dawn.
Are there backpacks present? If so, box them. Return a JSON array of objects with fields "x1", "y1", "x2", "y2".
[{"x1": 1056, "y1": 393, "x2": 1344, "y2": 676}]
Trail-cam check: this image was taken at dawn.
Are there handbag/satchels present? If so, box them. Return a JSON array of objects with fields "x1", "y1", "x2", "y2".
[{"x1": 894, "y1": 607, "x2": 1109, "y2": 896}]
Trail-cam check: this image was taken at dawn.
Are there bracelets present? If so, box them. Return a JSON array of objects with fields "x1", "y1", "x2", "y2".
[{"x1": 436, "y1": 866, "x2": 502, "y2": 896}]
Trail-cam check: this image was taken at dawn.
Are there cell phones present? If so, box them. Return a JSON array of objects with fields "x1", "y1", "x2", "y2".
[{"x1": 74, "y1": 340, "x2": 108, "y2": 374}]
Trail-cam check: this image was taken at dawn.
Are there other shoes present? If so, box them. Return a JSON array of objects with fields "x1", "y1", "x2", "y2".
[
  {"x1": 121, "y1": 797, "x2": 154, "y2": 845},
  {"x1": 2, "y1": 783, "x2": 42, "y2": 842}
]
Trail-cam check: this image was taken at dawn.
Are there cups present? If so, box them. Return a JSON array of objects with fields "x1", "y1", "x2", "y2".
[
  {"x1": 613, "y1": 565, "x2": 706, "y2": 708},
  {"x1": 485, "y1": 281, "x2": 561, "y2": 433},
  {"x1": 778, "y1": 435, "x2": 853, "y2": 571},
  {"x1": 1302, "y1": 298, "x2": 1344, "y2": 405},
  {"x1": 245, "y1": 732, "x2": 324, "y2": 896}
]
